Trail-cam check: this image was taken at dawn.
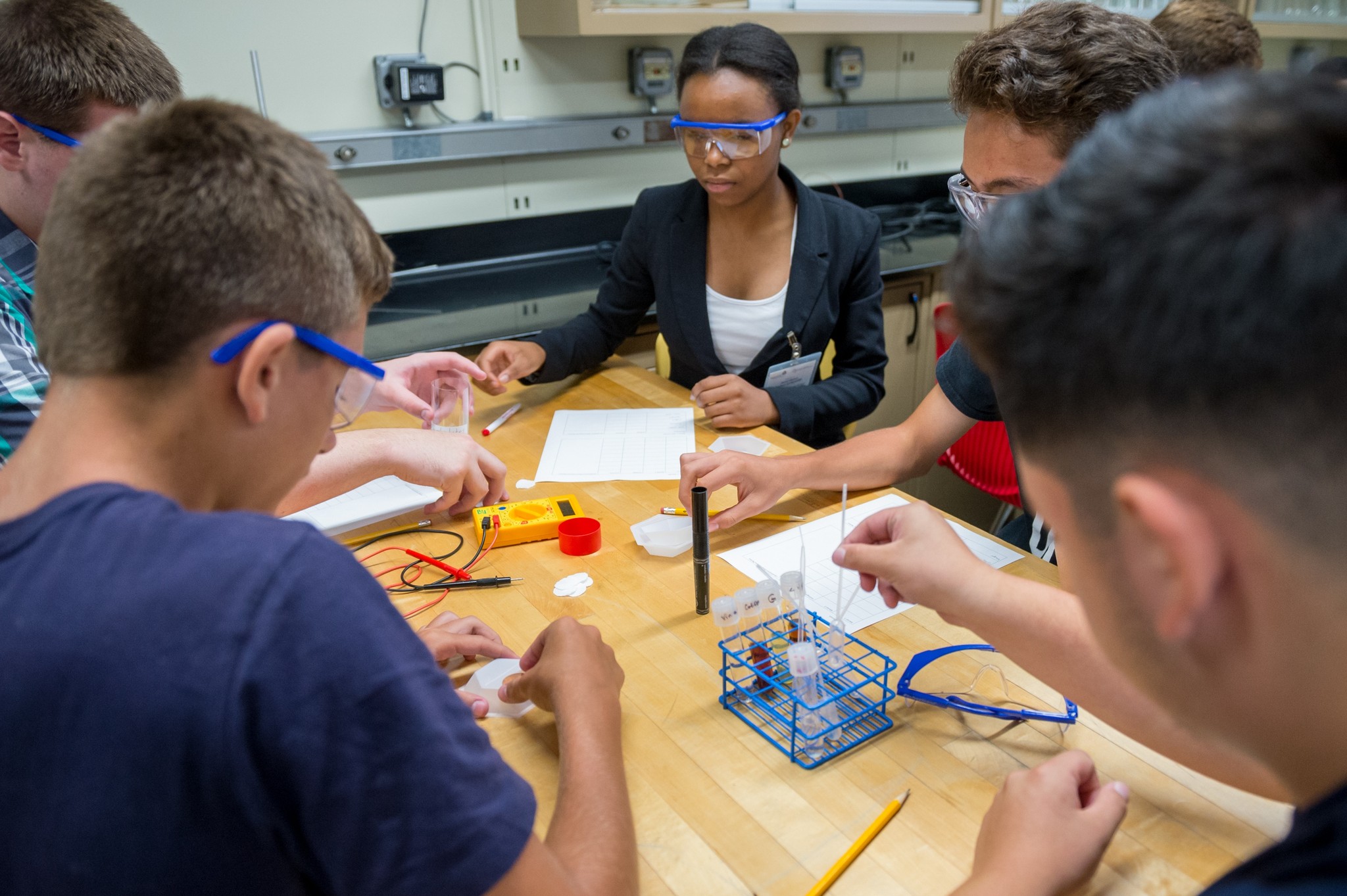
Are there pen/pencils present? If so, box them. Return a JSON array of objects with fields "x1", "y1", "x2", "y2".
[
  {"x1": 481, "y1": 401, "x2": 524, "y2": 436},
  {"x1": 806, "y1": 787, "x2": 912, "y2": 896},
  {"x1": 660, "y1": 507, "x2": 808, "y2": 521},
  {"x1": 335, "y1": 518, "x2": 431, "y2": 548}
]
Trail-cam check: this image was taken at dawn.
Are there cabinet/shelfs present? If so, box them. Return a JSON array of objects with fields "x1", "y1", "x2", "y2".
[{"x1": 514, "y1": 0, "x2": 1347, "y2": 44}]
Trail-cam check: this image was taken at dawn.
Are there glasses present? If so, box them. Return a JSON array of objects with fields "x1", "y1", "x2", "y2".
[
  {"x1": 946, "y1": 173, "x2": 1029, "y2": 228},
  {"x1": 670, "y1": 112, "x2": 785, "y2": 160},
  {"x1": 897, "y1": 643, "x2": 1080, "y2": 723},
  {"x1": 212, "y1": 320, "x2": 384, "y2": 429}
]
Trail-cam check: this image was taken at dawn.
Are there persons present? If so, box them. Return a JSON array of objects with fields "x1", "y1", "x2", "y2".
[
  {"x1": 473, "y1": 22, "x2": 890, "y2": 451},
  {"x1": 832, "y1": 71, "x2": 1347, "y2": 896},
  {"x1": 0, "y1": 0, "x2": 508, "y2": 517},
  {"x1": 679, "y1": 0, "x2": 1178, "y2": 565},
  {"x1": 1150, "y1": 0, "x2": 1264, "y2": 80},
  {"x1": 0, "y1": 96, "x2": 640, "y2": 896}
]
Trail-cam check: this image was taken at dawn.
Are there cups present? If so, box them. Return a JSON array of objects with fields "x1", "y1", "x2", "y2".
[{"x1": 430, "y1": 377, "x2": 470, "y2": 433}]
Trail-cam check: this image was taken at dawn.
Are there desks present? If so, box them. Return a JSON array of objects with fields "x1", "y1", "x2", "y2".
[{"x1": 328, "y1": 350, "x2": 1297, "y2": 896}]
